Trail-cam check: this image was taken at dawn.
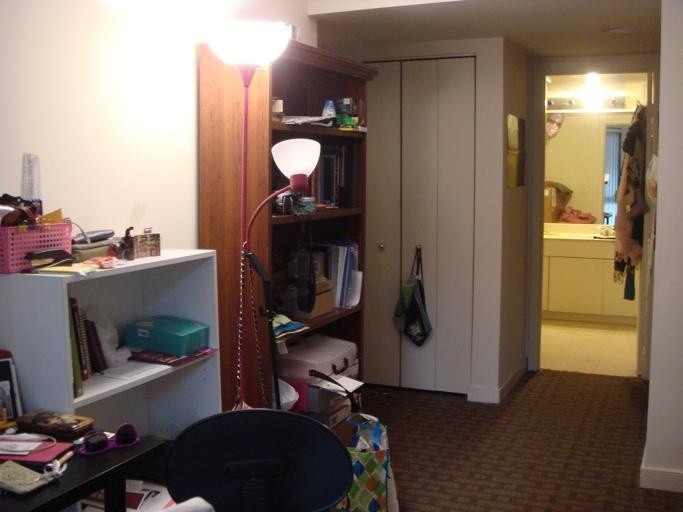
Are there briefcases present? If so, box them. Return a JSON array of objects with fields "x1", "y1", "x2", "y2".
[{"x1": 275, "y1": 333, "x2": 357, "y2": 384}]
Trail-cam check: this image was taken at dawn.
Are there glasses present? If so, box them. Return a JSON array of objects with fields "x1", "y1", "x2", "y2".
[{"x1": 77, "y1": 422, "x2": 141, "y2": 455}]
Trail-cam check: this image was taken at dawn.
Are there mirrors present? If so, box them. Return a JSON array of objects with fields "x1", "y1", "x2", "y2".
[{"x1": 601, "y1": 123, "x2": 632, "y2": 225}]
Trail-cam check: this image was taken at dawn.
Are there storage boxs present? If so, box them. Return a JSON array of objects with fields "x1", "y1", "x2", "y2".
[{"x1": 124, "y1": 314, "x2": 208, "y2": 357}]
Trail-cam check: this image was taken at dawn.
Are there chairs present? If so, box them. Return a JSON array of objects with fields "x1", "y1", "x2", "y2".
[{"x1": 166, "y1": 410, "x2": 353, "y2": 511}]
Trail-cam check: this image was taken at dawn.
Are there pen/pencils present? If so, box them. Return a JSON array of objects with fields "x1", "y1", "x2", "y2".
[
  {"x1": 0, "y1": 436, "x2": 48, "y2": 442},
  {"x1": 45, "y1": 450, "x2": 75, "y2": 472}
]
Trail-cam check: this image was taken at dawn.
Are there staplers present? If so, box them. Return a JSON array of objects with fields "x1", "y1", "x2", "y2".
[{"x1": 21, "y1": 249, "x2": 75, "y2": 273}]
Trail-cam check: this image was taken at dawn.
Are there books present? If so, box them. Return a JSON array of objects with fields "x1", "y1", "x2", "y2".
[
  {"x1": 68, "y1": 298, "x2": 108, "y2": 399},
  {"x1": 308, "y1": 140, "x2": 363, "y2": 308}
]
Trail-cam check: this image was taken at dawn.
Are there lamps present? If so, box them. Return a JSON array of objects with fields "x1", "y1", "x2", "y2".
[{"x1": 208, "y1": 19, "x2": 320, "y2": 412}]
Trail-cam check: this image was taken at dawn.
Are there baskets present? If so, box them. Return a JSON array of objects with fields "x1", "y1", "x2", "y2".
[{"x1": 0, "y1": 223, "x2": 73, "y2": 274}]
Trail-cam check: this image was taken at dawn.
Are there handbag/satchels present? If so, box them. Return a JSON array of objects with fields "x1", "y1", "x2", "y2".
[
  {"x1": 334, "y1": 413, "x2": 399, "y2": 512},
  {"x1": 622, "y1": 190, "x2": 651, "y2": 220},
  {"x1": 393, "y1": 277, "x2": 433, "y2": 347}
]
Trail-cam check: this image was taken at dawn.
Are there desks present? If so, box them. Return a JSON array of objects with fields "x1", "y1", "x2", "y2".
[{"x1": 0, "y1": 430, "x2": 166, "y2": 512}]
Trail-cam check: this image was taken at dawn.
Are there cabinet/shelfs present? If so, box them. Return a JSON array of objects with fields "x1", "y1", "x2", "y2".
[
  {"x1": 364, "y1": 38, "x2": 531, "y2": 404},
  {"x1": 540, "y1": 255, "x2": 640, "y2": 327},
  {"x1": 0, "y1": 247, "x2": 222, "y2": 443},
  {"x1": 195, "y1": 39, "x2": 379, "y2": 443}
]
